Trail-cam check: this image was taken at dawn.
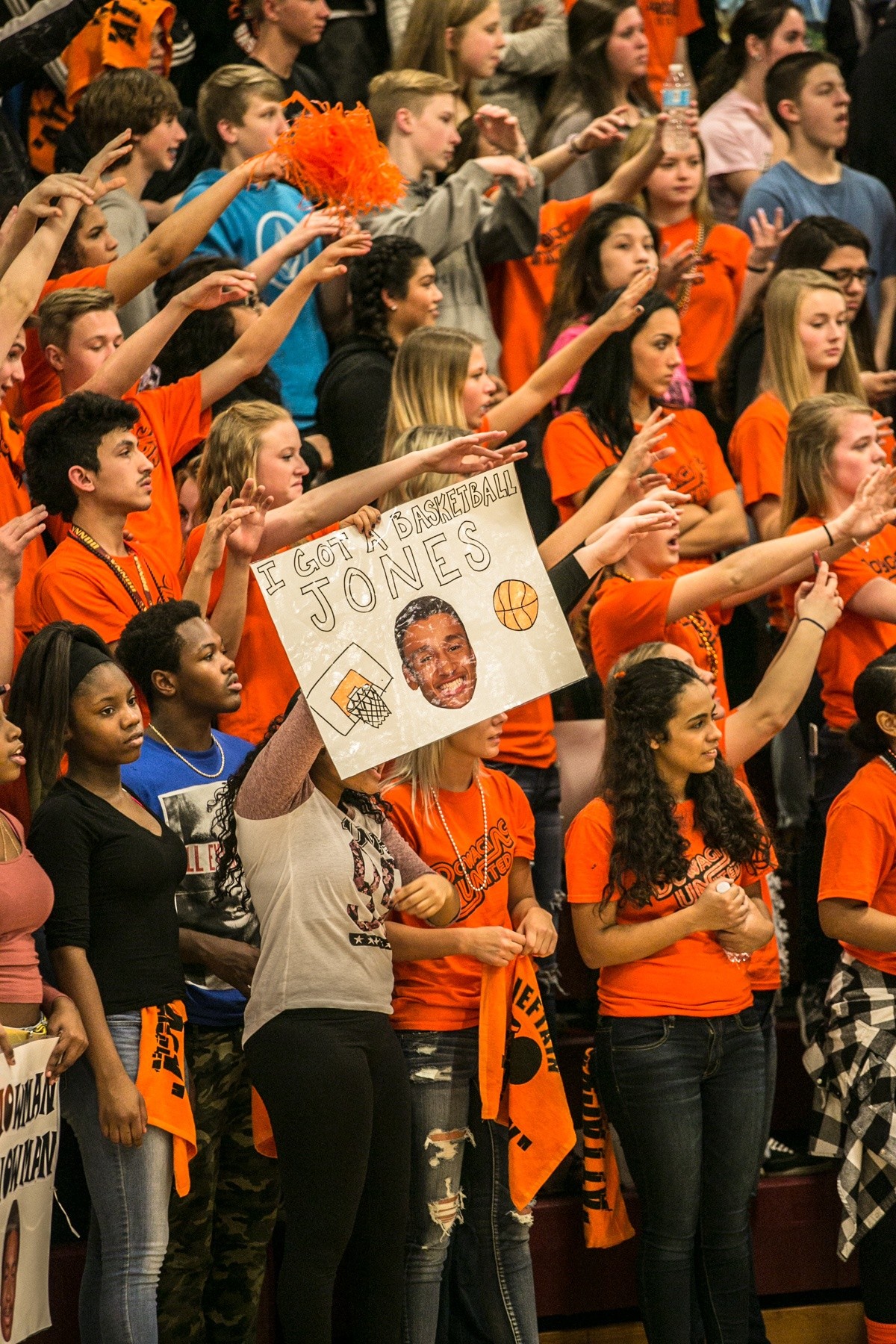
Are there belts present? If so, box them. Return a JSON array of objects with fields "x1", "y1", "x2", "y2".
[{"x1": 1, "y1": 1017, "x2": 51, "y2": 1049}]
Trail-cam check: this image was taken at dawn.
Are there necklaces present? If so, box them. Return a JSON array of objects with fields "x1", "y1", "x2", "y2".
[
  {"x1": 0, "y1": 816, "x2": 19, "y2": 861},
  {"x1": 426, "y1": 773, "x2": 488, "y2": 892},
  {"x1": 887, "y1": 746, "x2": 896, "y2": 759},
  {"x1": 615, "y1": 571, "x2": 718, "y2": 683},
  {"x1": 149, "y1": 722, "x2": 225, "y2": 778},
  {"x1": 672, "y1": 219, "x2": 711, "y2": 318},
  {"x1": 879, "y1": 754, "x2": 896, "y2": 774}
]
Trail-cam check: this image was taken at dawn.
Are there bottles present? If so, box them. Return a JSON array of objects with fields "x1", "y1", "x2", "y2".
[
  {"x1": 659, "y1": 64, "x2": 692, "y2": 155},
  {"x1": 716, "y1": 881, "x2": 753, "y2": 963}
]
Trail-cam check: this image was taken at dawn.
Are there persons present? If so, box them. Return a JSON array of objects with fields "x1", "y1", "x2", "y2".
[
  {"x1": 0, "y1": 0, "x2": 896, "y2": 1344},
  {"x1": 394, "y1": 597, "x2": 476, "y2": 708}
]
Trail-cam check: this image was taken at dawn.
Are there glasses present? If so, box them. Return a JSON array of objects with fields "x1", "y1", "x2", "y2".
[
  {"x1": 818, "y1": 267, "x2": 879, "y2": 288},
  {"x1": 227, "y1": 295, "x2": 262, "y2": 310}
]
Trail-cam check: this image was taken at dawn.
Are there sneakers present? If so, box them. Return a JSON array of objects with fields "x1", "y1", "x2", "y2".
[{"x1": 759, "y1": 1136, "x2": 834, "y2": 1176}]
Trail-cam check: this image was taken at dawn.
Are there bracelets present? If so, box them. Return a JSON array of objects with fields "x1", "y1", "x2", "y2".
[
  {"x1": 565, "y1": 132, "x2": 587, "y2": 159},
  {"x1": 822, "y1": 525, "x2": 834, "y2": 546},
  {"x1": 746, "y1": 264, "x2": 768, "y2": 273},
  {"x1": 853, "y1": 538, "x2": 871, "y2": 552},
  {"x1": 571, "y1": 137, "x2": 589, "y2": 154},
  {"x1": 799, "y1": 617, "x2": 828, "y2": 634}
]
